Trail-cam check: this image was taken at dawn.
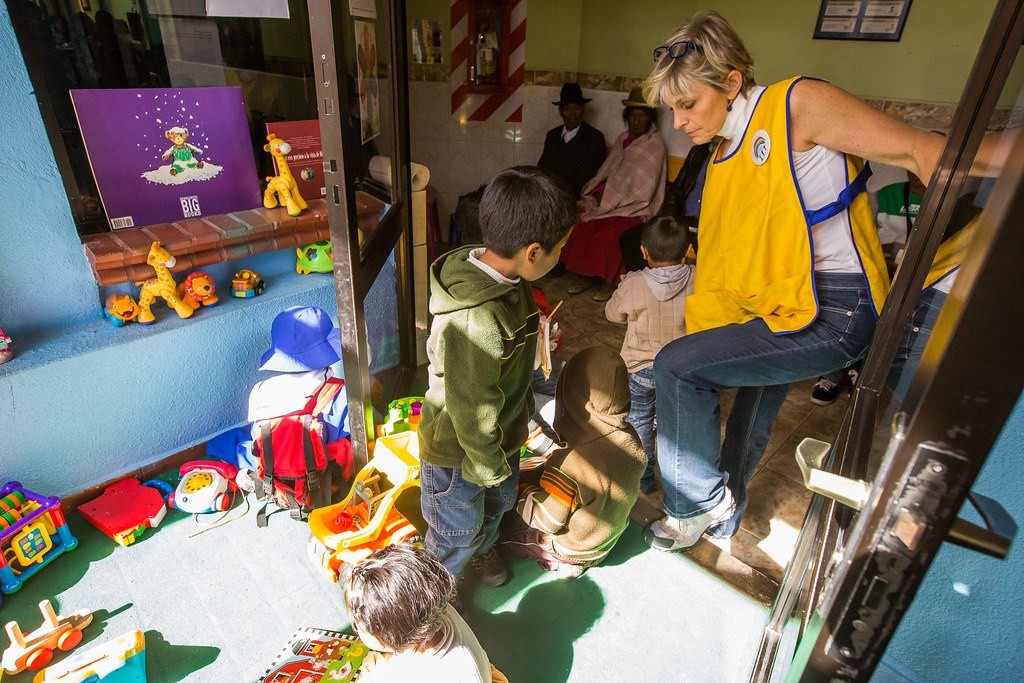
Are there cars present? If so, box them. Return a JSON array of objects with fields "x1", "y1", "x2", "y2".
[{"x1": 230, "y1": 269, "x2": 266, "y2": 297}]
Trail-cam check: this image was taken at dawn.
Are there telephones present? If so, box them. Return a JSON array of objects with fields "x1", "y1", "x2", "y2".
[{"x1": 167, "y1": 459, "x2": 238, "y2": 514}]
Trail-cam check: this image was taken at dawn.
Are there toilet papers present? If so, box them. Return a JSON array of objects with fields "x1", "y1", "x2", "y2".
[{"x1": 369, "y1": 155, "x2": 430, "y2": 192}]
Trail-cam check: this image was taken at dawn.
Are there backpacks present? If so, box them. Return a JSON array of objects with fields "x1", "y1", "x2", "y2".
[{"x1": 249, "y1": 378, "x2": 355, "y2": 527}]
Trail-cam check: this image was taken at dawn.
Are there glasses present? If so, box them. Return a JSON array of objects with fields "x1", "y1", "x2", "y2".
[{"x1": 652, "y1": 40, "x2": 703, "y2": 62}]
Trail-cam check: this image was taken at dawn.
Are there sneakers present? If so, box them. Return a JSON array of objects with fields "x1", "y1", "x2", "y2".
[
  {"x1": 469, "y1": 545, "x2": 508, "y2": 586},
  {"x1": 810, "y1": 378, "x2": 839, "y2": 406},
  {"x1": 642, "y1": 471, "x2": 737, "y2": 554},
  {"x1": 448, "y1": 574, "x2": 471, "y2": 625}
]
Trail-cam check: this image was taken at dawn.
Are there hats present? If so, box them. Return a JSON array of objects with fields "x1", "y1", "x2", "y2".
[
  {"x1": 257, "y1": 306, "x2": 343, "y2": 373},
  {"x1": 621, "y1": 87, "x2": 660, "y2": 108},
  {"x1": 550, "y1": 83, "x2": 593, "y2": 105}
]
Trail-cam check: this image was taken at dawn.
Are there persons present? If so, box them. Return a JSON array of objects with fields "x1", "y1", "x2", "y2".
[
  {"x1": 968, "y1": 123, "x2": 1022, "y2": 179},
  {"x1": 568, "y1": 86, "x2": 667, "y2": 302},
  {"x1": 810, "y1": 130, "x2": 948, "y2": 405},
  {"x1": 247, "y1": 306, "x2": 355, "y2": 528},
  {"x1": 604, "y1": 214, "x2": 696, "y2": 494},
  {"x1": 642, "y1": 9, "x2": 949, "y2": 553},
  {"x1": 338, "y1": 542, "x2": 492, "y2": 683},
  {"x1": 536, "y1": 83, "x2": 608, "y2": 201},
  {"x1": 417, "y1": 164, "x2": 577, "y2": 590}
]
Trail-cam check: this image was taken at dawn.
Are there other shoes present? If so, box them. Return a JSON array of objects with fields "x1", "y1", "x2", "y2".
[
  {"x1": 567, "y1": 281, "x2": 595, "y2": 294},
  {"x1": 547, "y1": 264, "x2": 569, "y2": 278},
  {"x1": 591, "y1": 286, "x2": 617, "y2": 302}
]
